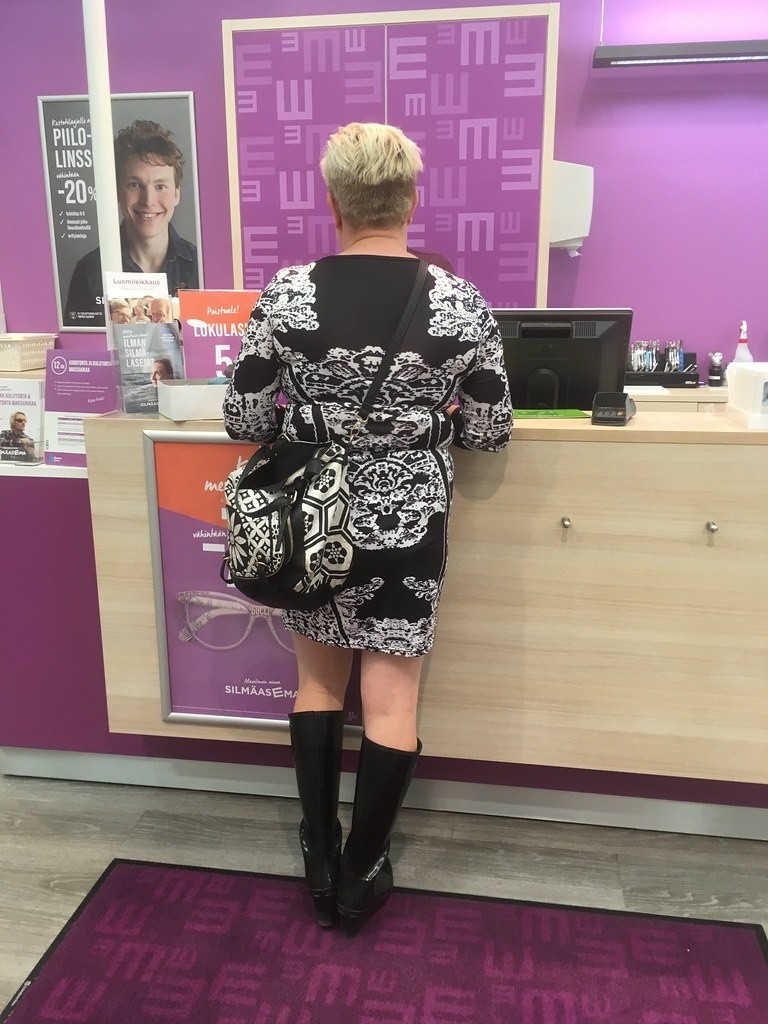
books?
[113,322,185,414]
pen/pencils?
[684,363,698,372]
[664,339,683,372]
[630,340,660,372]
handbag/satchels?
[220,440,354,611]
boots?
[289,710,343,927]
[336,730,422,917]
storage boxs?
[157,377,232,421]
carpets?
[0,857,767,1024]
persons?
[108,295,171,324]
[150,358,174,389]
[222,121,513,939]
[0,412,34,462]
[63,120,199,326]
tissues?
[548,161,594,256]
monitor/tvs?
[490,308,633,411]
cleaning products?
[727,320,754,362]
